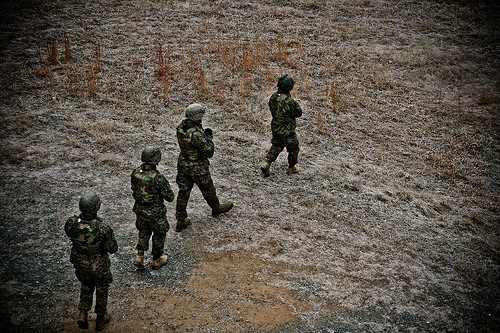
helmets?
[79,193,101,213]
[141,148,161,164]
[185,103,205,121]
[277,74,294,89]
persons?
[64,191,118,331]
[175,104,233,231]
[260,75,303,177]
[130,146,174,269]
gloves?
[204,128,212,136]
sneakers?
[151,255,168,269]
[176,218,191,232]
[95,314,110,328]
[212,202,233,217]
[133,255,144,267]
[77,310,89,330]
[287,167,301,175]
[261,162,271,177]
